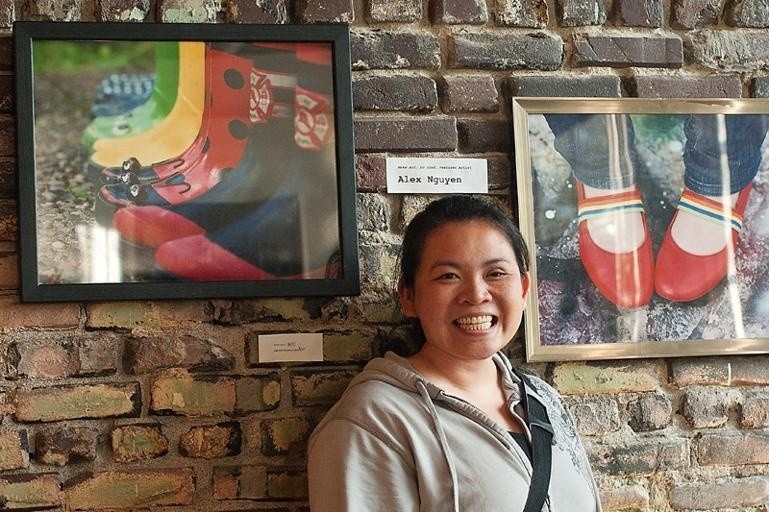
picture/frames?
[506,92,769,367]
[9,19,357,306]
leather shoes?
[655,183,752,303]
[576,180,655,307]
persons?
[306,196,601,512]
[540,114,769,307]
